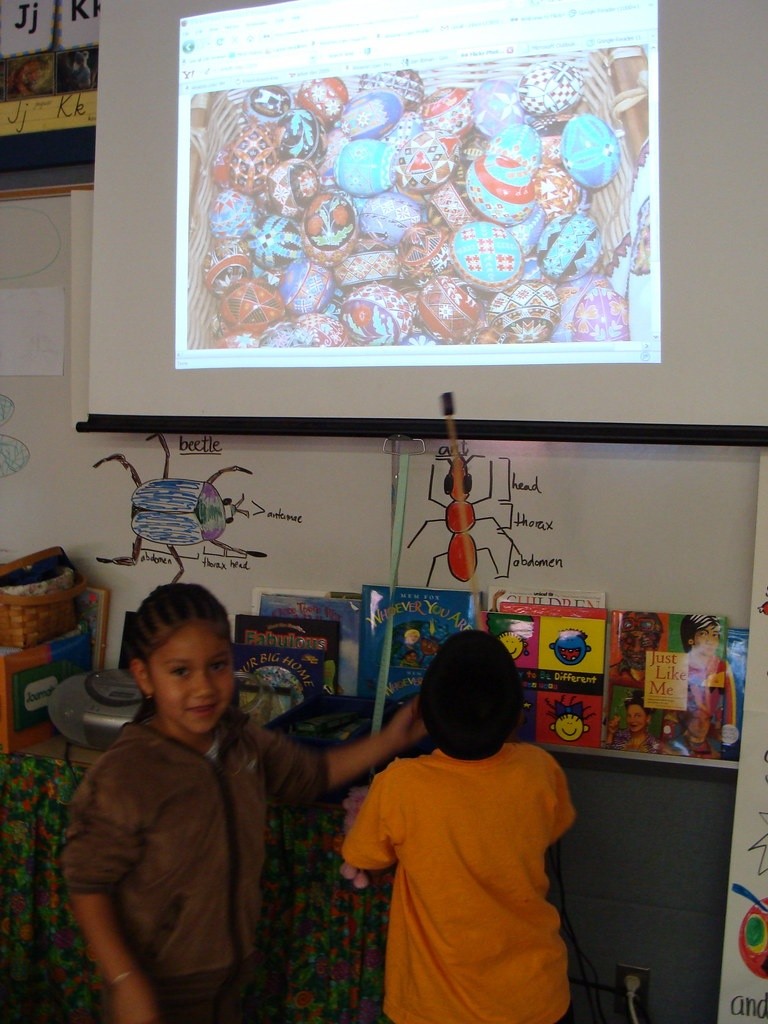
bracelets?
[105,969,137,989]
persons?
[341,629,576,1024]
[63,582,430,1023]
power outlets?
[613,964,651,1019]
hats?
[417,629,524,761]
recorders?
[48,667,143,753]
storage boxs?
[0,631,93,754]
[262,693,395,804]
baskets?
[0,546,90,648]
